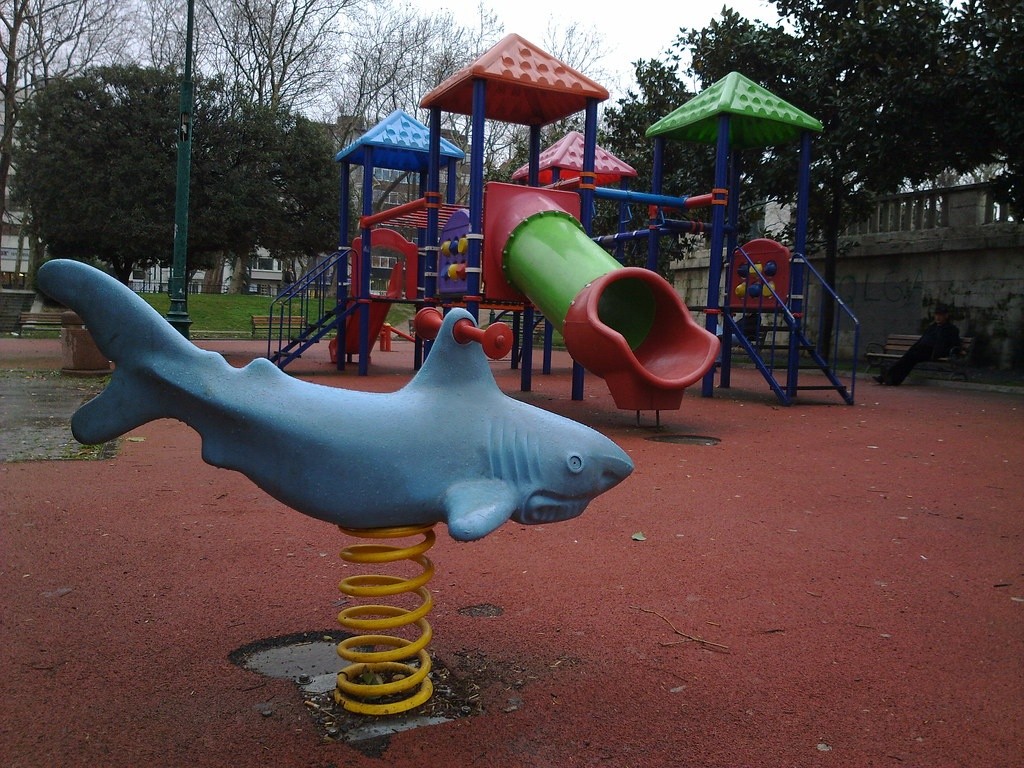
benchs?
[510,320,545,343]
[251,315,307,338]
[864,333,978,382]
[731,329,767,368]
[15,312,63,336]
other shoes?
[718,336,723,342]
[715,362,721,367]
[871,376,899,385]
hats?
[932,305,949,314]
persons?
[871,306,960,387]
[281,267,295,298]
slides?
[326,264,404,363]
[495,193,720,411]
